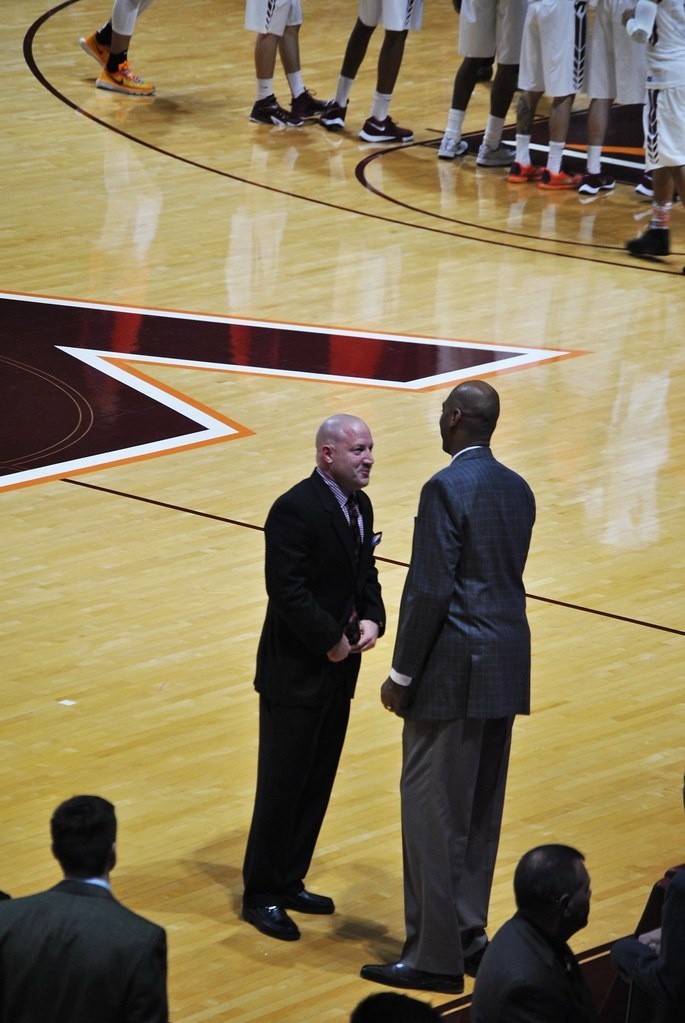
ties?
[347,499,362,541]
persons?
[240,413,389,941]
[244,0,333,127]
[0,795,169,1023]
[319,0,424,143]
[350,992,444,1023]
[469,842,599,1023]
[437,0,685,257]
[610,776,685,1023]
[359,379,537,994]
[80,0,156,97]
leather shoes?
[464,942,490,977]
[360,961,465,994]
[243,903,301,941]
[286,890,335,914]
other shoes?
[627,228,671,257]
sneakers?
[95,60,156,95]
[673,192,681,202]
[319,102,347,129]
[291,87,348,121]
[476,144,517,166]
[540,168,583,190]
[249,94,304,127]
[577,171,616,194]
[79,31,112,67]
[635,174,655,197]
[438,136,468,157]
[508,162,546,183]
[359,116,414,143]
